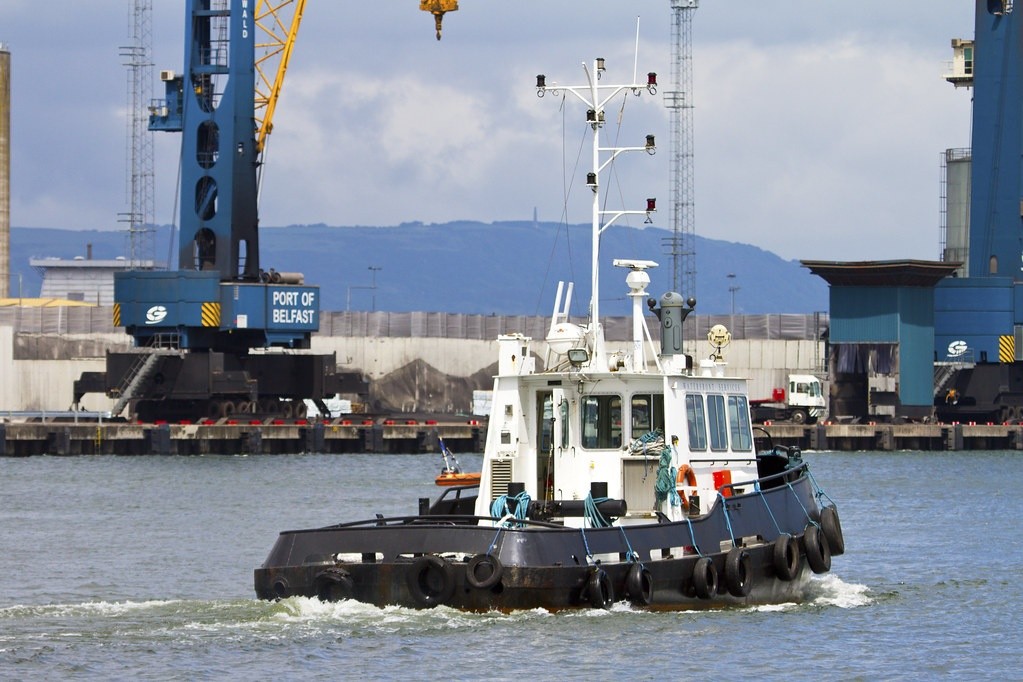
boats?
[254,57,847,613]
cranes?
[255,1,459,153]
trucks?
[749,374,827,424]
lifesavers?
[676,464,697,510]
